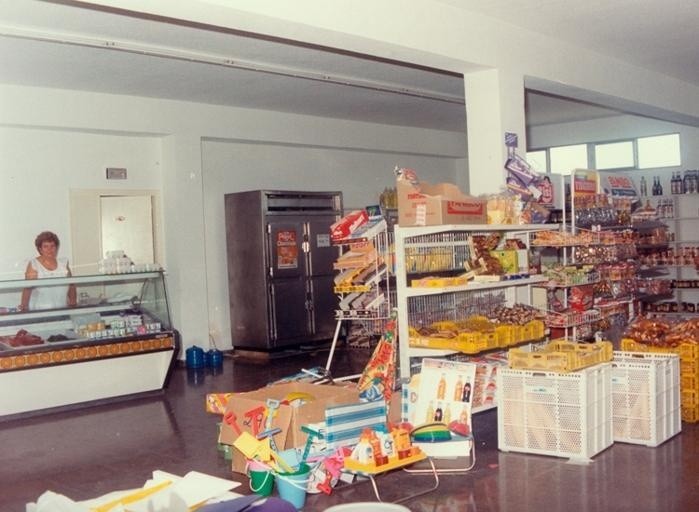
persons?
[18,232,77,315]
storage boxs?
[621,336,698,423]
[610,351,684,447]
[218,380,359,479]
[394,177,489,226]
[498,358,612,458]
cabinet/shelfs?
[0,270,177,420]
[572,224,676,350]
[224,188,350,352]
[334,170,569,419]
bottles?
[672,168,698,198]
[656,177,663,195]
[652,179,660,196]
[640,176,647,195]
[654,199,674,222]
